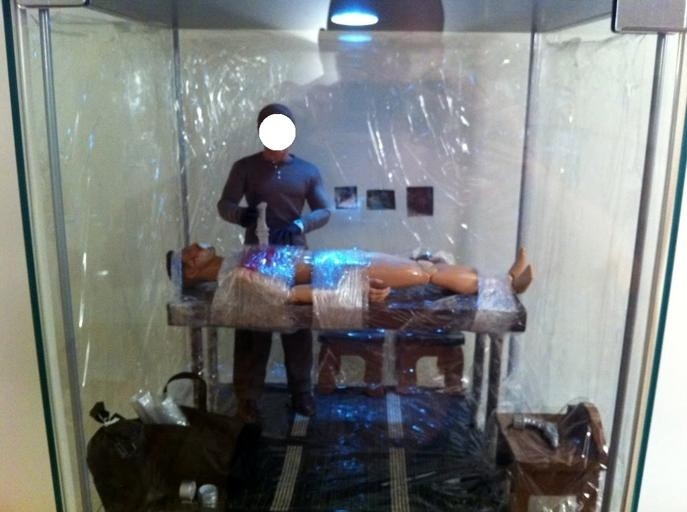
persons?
[255,1,490,130]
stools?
[315,328,470,399]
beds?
[168,284,528,438]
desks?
[494,399,609,512]
